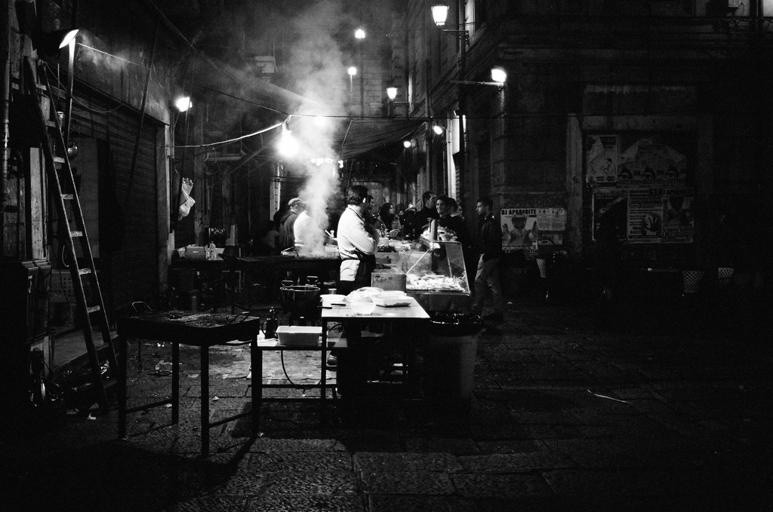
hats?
[288,198,303,205]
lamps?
[56,26,80,49]
[430,0,470,46]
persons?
[616,169,633,184]
[584,133,608,182]
[643,214,660,235]
[502,214,537,246]
[269,185,512,326]
[653,127,738,331]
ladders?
[25,57,118,414]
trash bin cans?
[423,308,497,402]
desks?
[320,292,432,438]
[255,325,348,432]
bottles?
[209,241,216,259]
[261,307,279,338]
